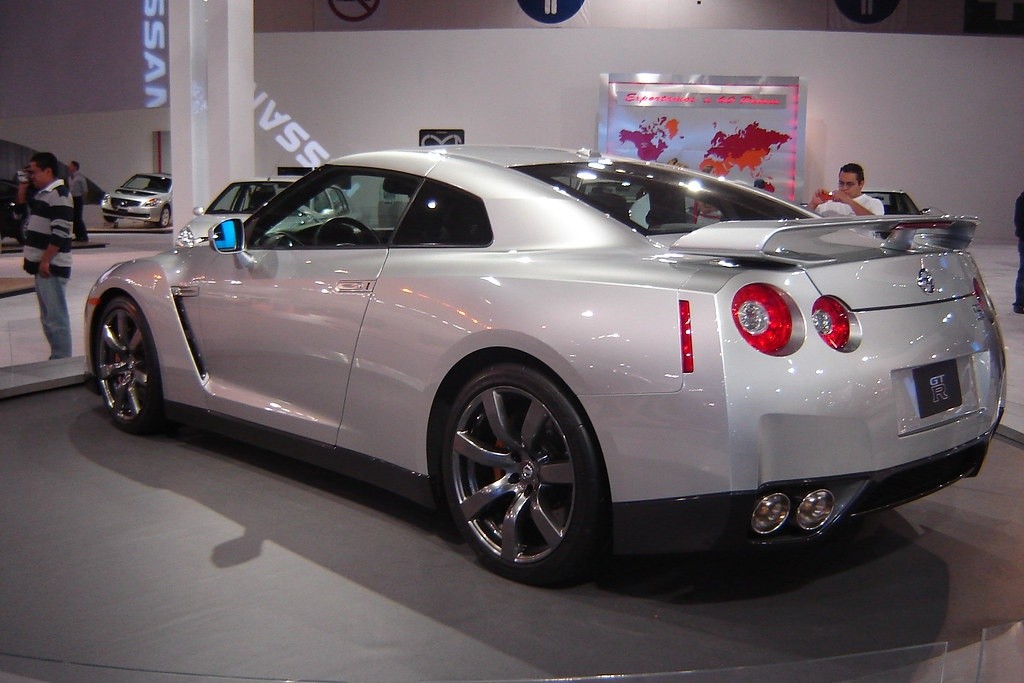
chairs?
[589,191,627,214]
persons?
[16,166,31,221]
[67,161,89,241]
[22,152,73,359]
[1013,190,1024,313]
[807,163,884,236]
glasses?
[838,182,858,187]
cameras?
[16,170,32,183]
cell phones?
[820,192,833,200]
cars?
[99,173,173,229]
[802,190,931,218]
[175,174,363,248]
[0,178,30,244]
[79,144,1009,583]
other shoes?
[1013,305,1024,314]
